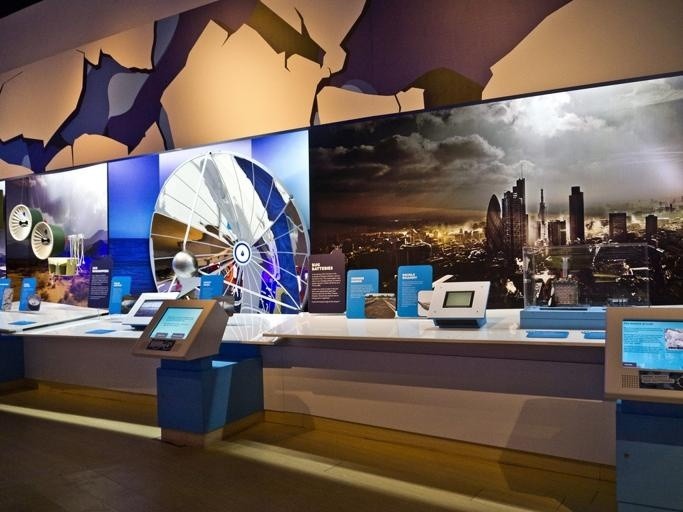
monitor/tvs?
[130,299,229,360]
[122,292,180,331]
[603,307,683,404]
